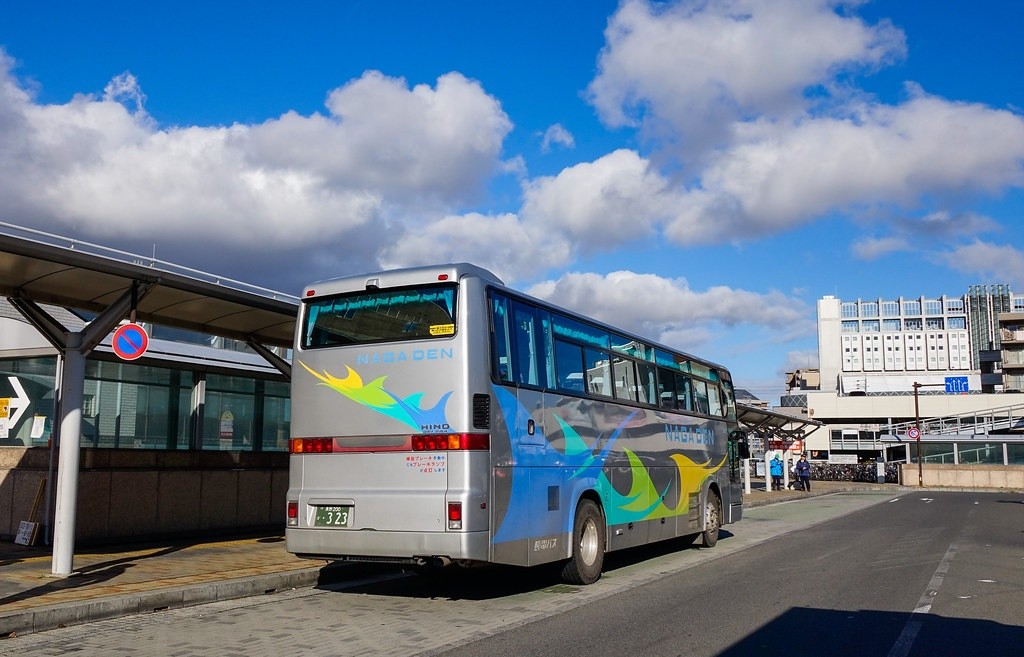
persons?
[770,454,782,491]
[796,453,810,492]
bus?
[282,262,748,586]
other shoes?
[773,484,811,492]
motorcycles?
[782,461,898,484]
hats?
[774,454,806,460]
